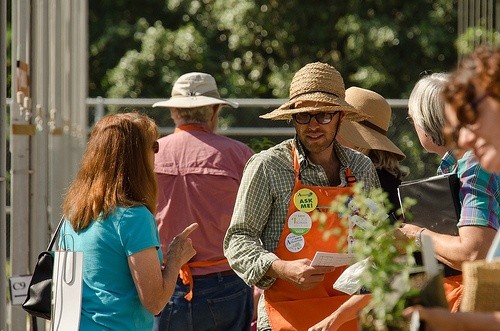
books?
[397,174,462,237]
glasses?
[448,93,490,133]
[150,141,159,153]
[406,116,414,125]
[291,111,338,125]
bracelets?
[415,229,422,248]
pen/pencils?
[391,212,405,228]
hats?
[337,86,406,159]
[259,62,367,121]
[152,72,239,108]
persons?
[335,86,406,227]
[59,112,199,331]
[151,72,256,331]
[307,46,500,331]
[222,62,381,331]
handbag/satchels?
[21,214,65,320]
